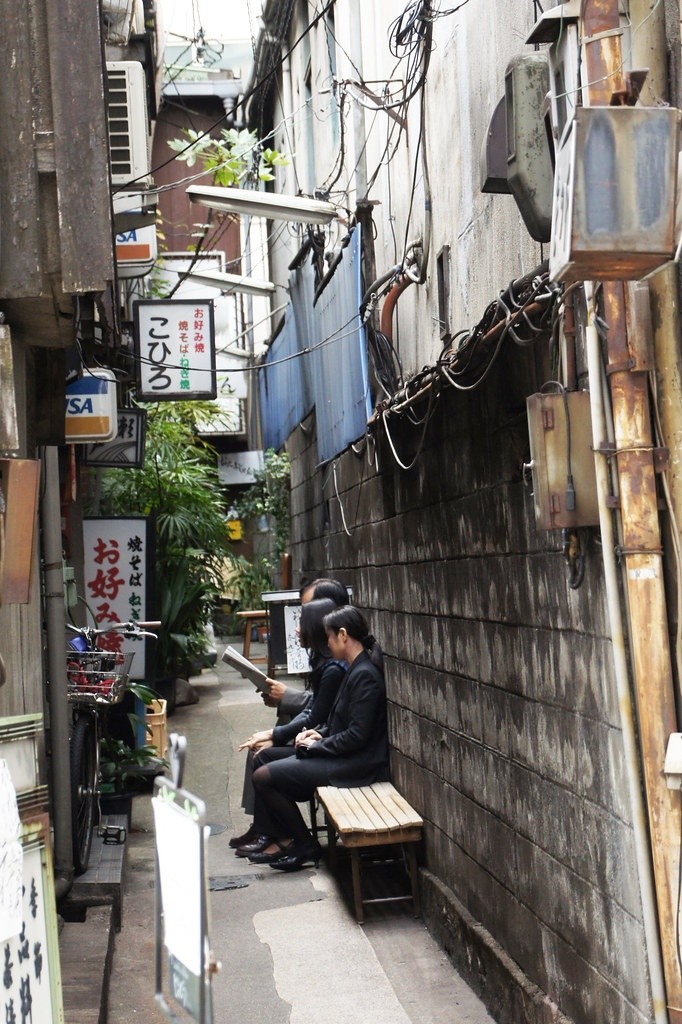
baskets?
[144,697,168,762]
[43,645,134,706]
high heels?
[249,837,297,861]
[269,840,323,872]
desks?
[236,609,269,677]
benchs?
[310,781,423,925]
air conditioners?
[106,59,151,189]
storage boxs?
[144,698,167,758]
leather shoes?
[235,834,268,857]
[229,825,263,847]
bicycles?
[62,618,163,874]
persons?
[240,604,387,876]
[234,598,351,862]
[229,577,350,858]
